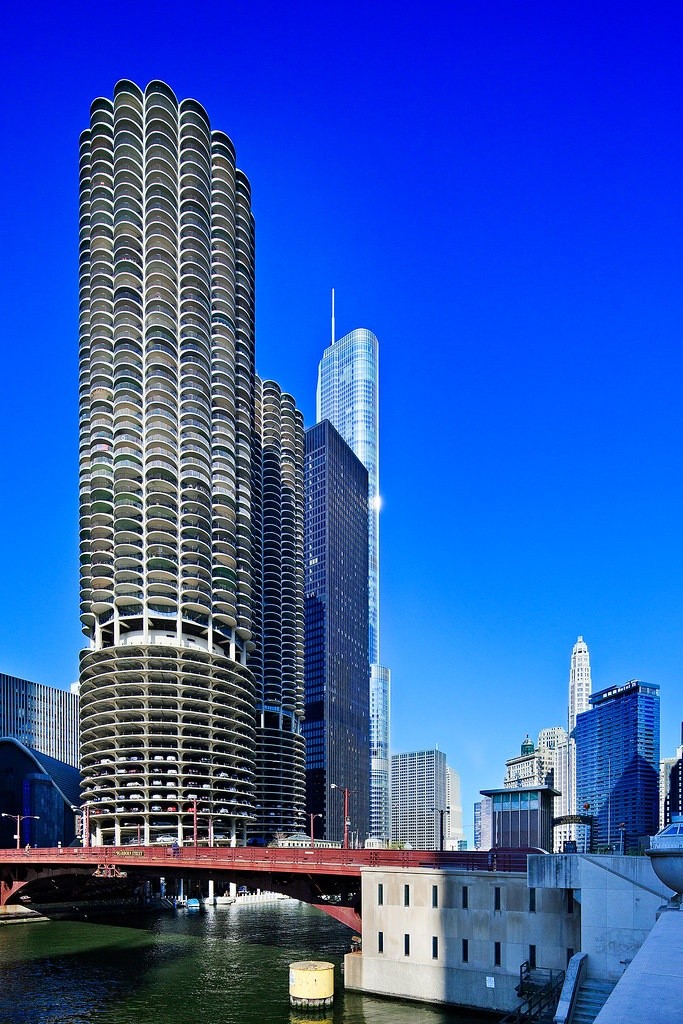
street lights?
[1,812,41,849]
[297,812,323,848]
[329,782,361,850]
[430,806,450,852]
[197,816,222,846]
[70,804,90,848]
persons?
[25,843,31,857]
[172,839,179,858]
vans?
[488,846,551,872]
